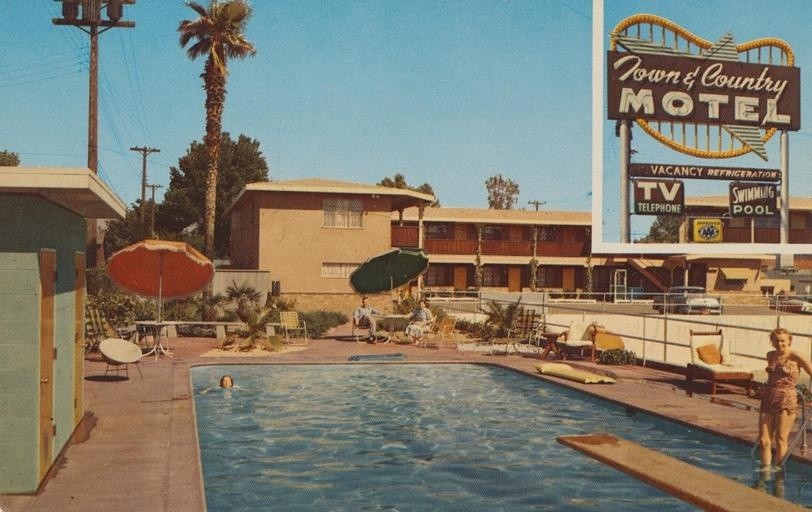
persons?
[199,374,245,396]
[734,466,787,503]
[406,301,436,345]
[751,328,812,471]
[354,295,382,344]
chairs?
[538,321,573,360]
[352,310,377,343]
[687,330,754,403]
[491,309,544,360]
[565,321,596,361]
[99,338,144,382]
[279,311,307,346]
[412,313,457,351]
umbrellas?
[102,236,218,324]
[347,247,432,316]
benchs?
[263,323,280,336]
[163,321,245,339]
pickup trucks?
[653,284,722,316]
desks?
[135,321,185,363]
[377,315,410,344]
